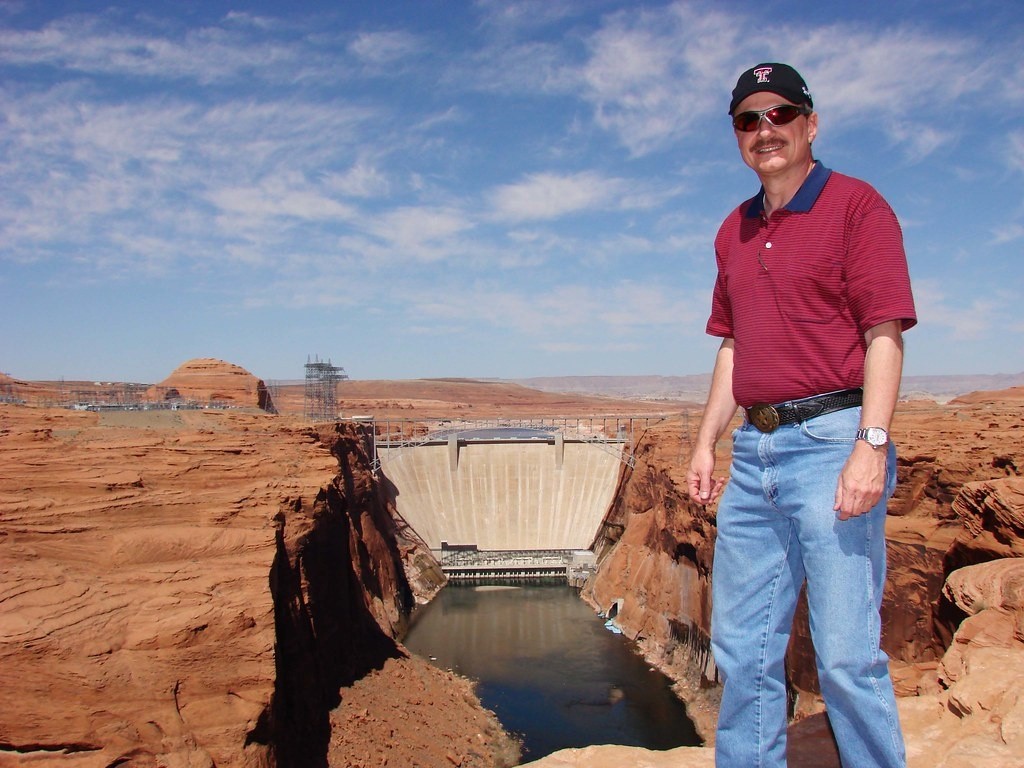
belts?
[742,387,863,433]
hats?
[728,63,813,115]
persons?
[686,62,917,768]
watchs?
[855,427,890,450]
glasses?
[732,102,811,132]
